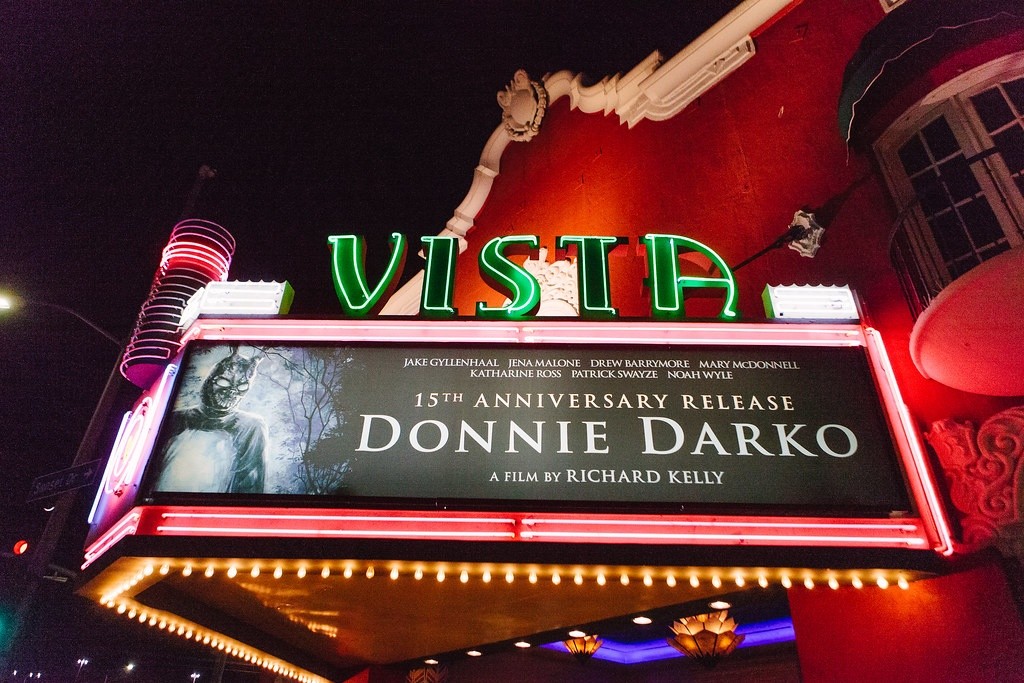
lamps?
[563,635,604,664]
[667,610,746,671]
